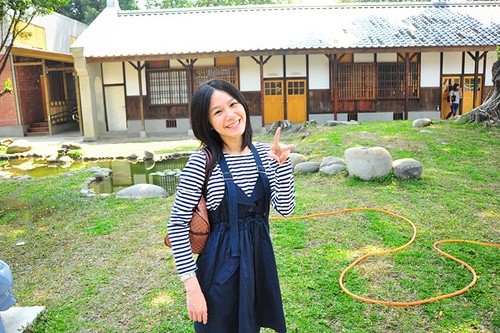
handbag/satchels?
[447,90,455,102]
[164,148,210,254]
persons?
[166,76,296,333]
[446,83,462,119]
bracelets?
[181,271,196,282]
